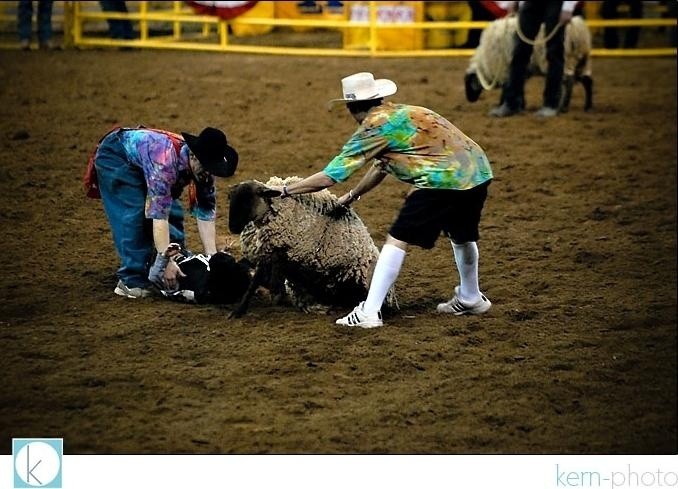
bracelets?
[349,189,362,202]
[282,186,288,195]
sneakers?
[488,102,513,116]
[437,284,492,315]
[335,301,384,329]
[114,278,151,299]
[533,106,556,117]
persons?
[266,71,495,329]
[599,0,641,47]
[489,1,580,117]
[17,0,53,49]
[147,242,254,305]
[100,0,135,51]
[83,124,239,301]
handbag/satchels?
[82,127,119,198]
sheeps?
[223,173,404,321]
[461,12,595,113]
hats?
[206,253,250,304]
[327,72,397,112]
[181,127,238,177]
